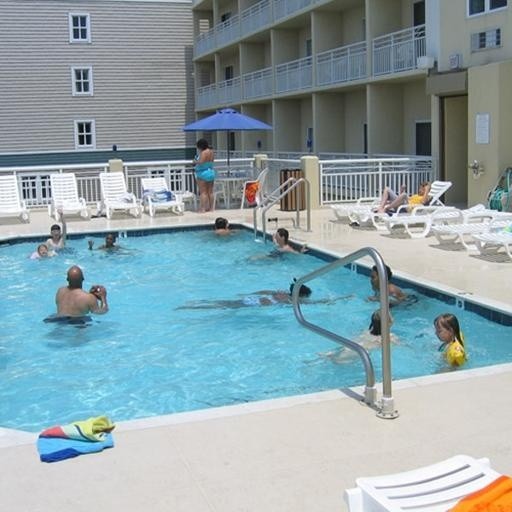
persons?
[173,276,356,309]
[193,138,216,214]
[87,232,134,258]
[246,228,310,264]
[214,216,232,236]
[370,182,432,214]
[37,243,57,259]
[433,313,468,372]
[313,308,402,364]
[363,263,424,315]
[44,207,67,251]
[42,265,109,346]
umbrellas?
[181,108,275,210]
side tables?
[174,190,197,211]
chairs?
[0,171,180,224]
[330,180,512,263]
[212,165,269,209]
